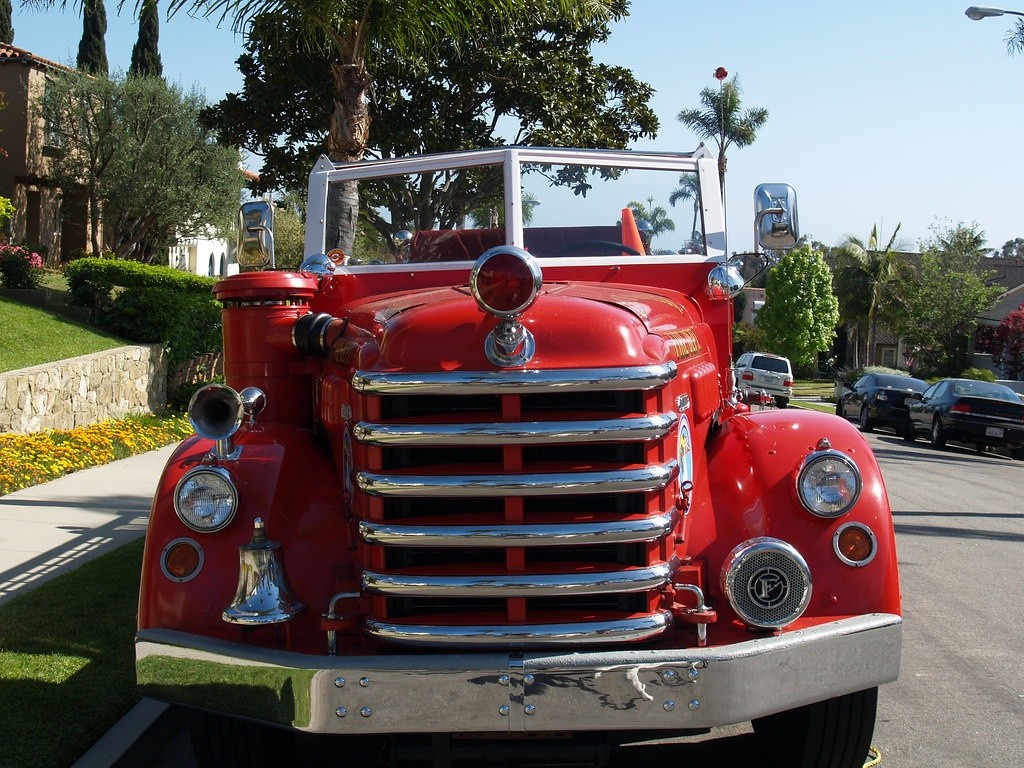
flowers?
[0,241,48,290]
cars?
[904,378,1024,458]
[133,144,901,768]
[835,373,930,442]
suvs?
[733,351,793,408]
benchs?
[410,226,623,264]
